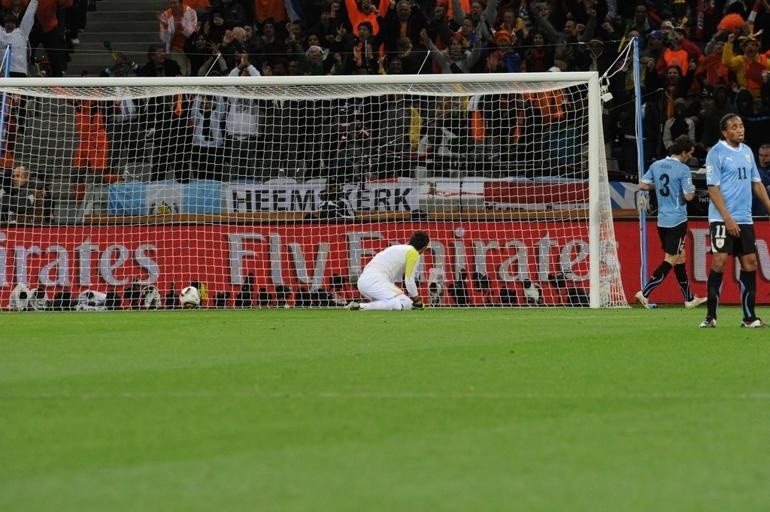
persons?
[347,231,429,310]
[0,1,770,215]
[634,133,707,309]
[694,112,770,327]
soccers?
[180,285,201,306]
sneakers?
[740,318,766,328]
[700,318,716,327]
[634,291,653,309]
[684,295,708,309]
